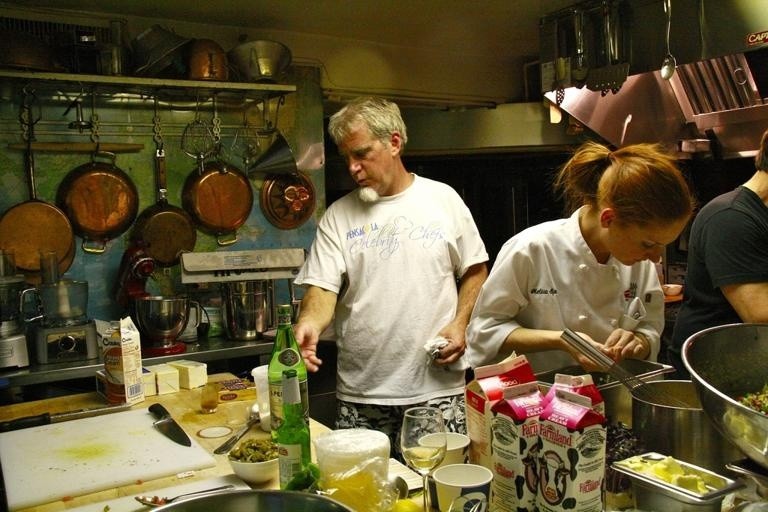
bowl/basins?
[681,321,767,469]
[130,23,198,80]
[229,39,292,81]
[227,451,277,485]
[663,283,682,296]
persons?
[288,93,490,480]
[664,130,766,381]
[462,143,694,369]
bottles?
[268,303,311,442]
[276,369,312,492]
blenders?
[111,246,189,357]
[17,280,100,365]
[0,250,29,370]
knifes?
[148,403,191,448]
[213,419,261,454]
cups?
[421,431,470,506]
[318,429,388,511]
[432,461,494,512]
[251,362,273,431]
[202,384,220,411]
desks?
[1,372,440,512]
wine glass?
[400,407,446,511]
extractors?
[666,52,757,116]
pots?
[0,141,78,283]
[630,378,751,484]
[181,149,254,247]
[57,150,139,253]
[134,149,196,267]
[145,488,356,510]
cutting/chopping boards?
[1,408,218,511]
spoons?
[139,483,234,507]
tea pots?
[179,301,200,343]
